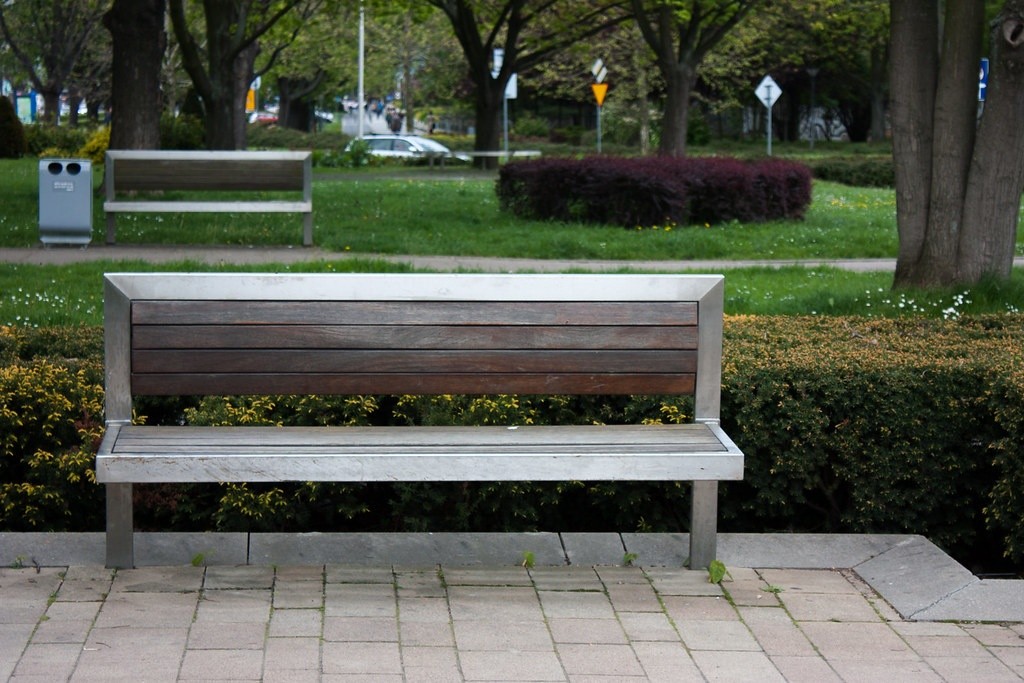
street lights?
[805,59,822,149]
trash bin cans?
[36,157,95,250]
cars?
[343,132,472,167]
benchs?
[103,150,314,247]
[414,150,542,170]
[94,272,749,567]
